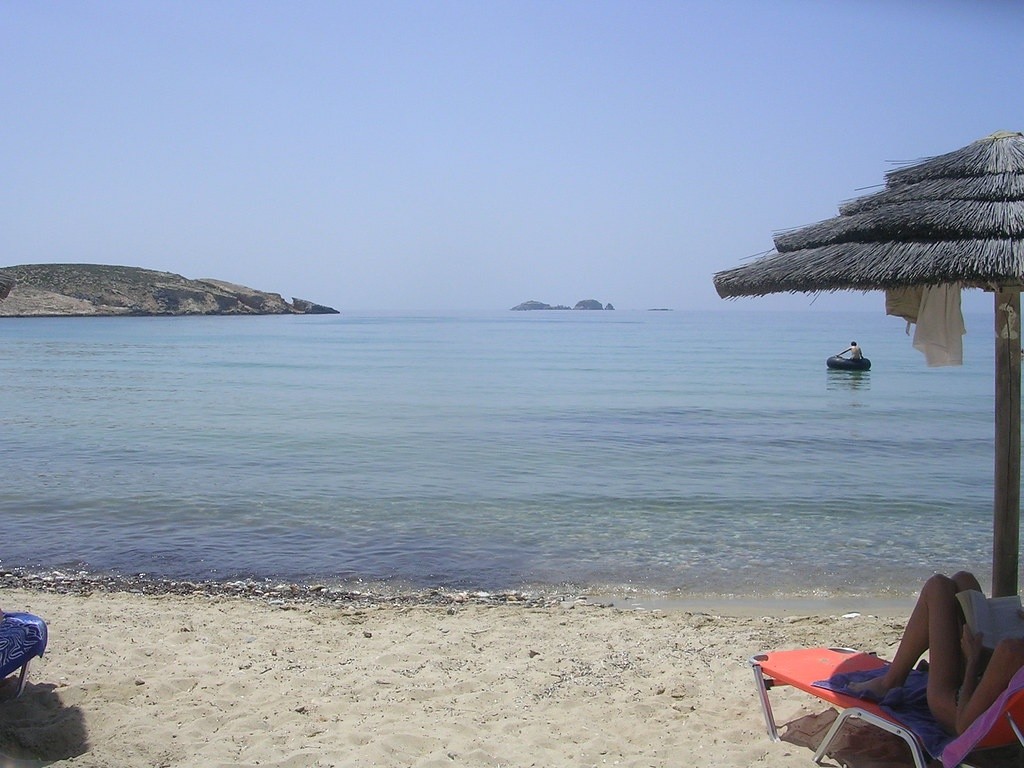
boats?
[826,355,872,372]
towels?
[942,665,1024,768]
[886,286,923,336]
[912,283,967,368]
[811,663,956,761]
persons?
[839,342,863,360]
[849,572,1024,735]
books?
[955,589,1024,648]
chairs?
[0,610,48,705]
[750,647,1024,768]
